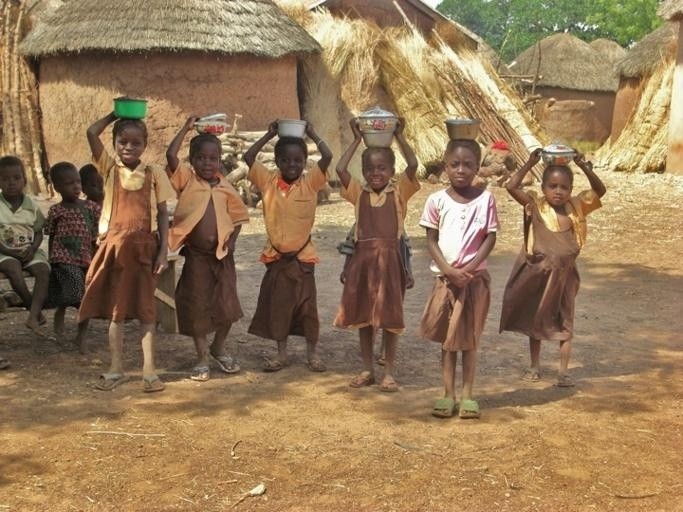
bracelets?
[315,140,322,147]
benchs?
[0,252,186,342]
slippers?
[556,374,575,388]
[259,355,289,371]
[305,359,326,372]
[348,369,374,388]
[189,366,210,381]
[458,399,480,419]
[207,350,240,373]
[430,398,456,418]
[142,376,164,392]
[520,367,543,382]
[94,372,129,391]
[379,378,397,392]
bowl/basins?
[275,119,306,139]
[443,119,481,140]
[193,120,227,135]
[539,145,576,166]
[113,98,148,120]
[357,106,397,148]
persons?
[418,138,502,418]
[75,94,170,392]
[242,118,333,371]
[44,161,98,356]
[78,163,104,219]
[0,155,51,338]
[497,146,606,390]
[337,215,414,366]
[335,116,418,392]
[164,114,250,382]
